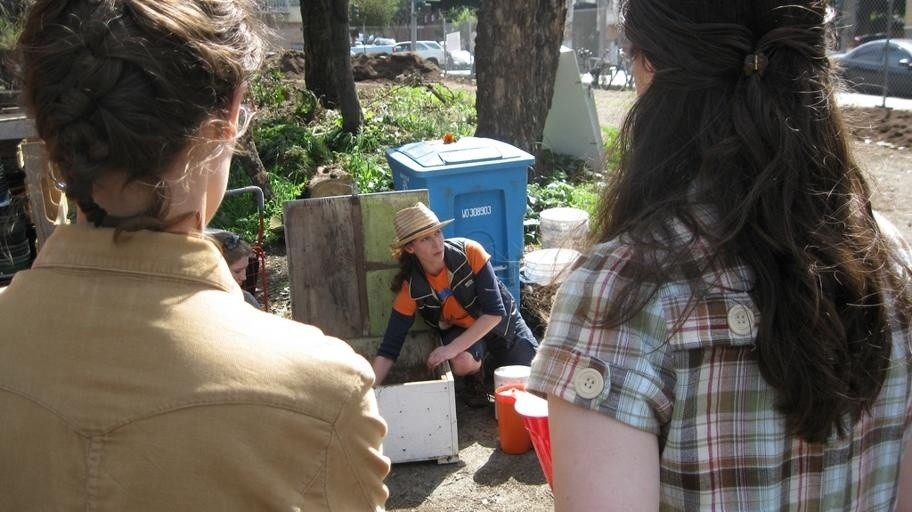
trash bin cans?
[386,136,535,313]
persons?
[205,229,251,290]
[371,201,540,408]
[523,0,912,512]
[0,0,391,512]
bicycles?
[584,48,635,89]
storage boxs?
[282,330,459,464]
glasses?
[222,233,240,253]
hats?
[389,202,456,249]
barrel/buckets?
[492,363,533,423]
[485,384,534,458]
[513,392,554,499]
[538,206,590,256]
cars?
[832,36,911,93]
[350,38,475,70]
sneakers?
[463,365,489,406]
